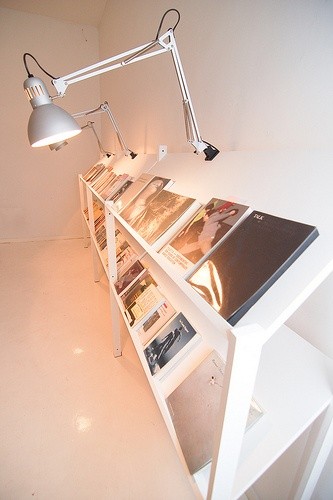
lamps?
[23,8,220,162]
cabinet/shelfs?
[78,152,333,500]
